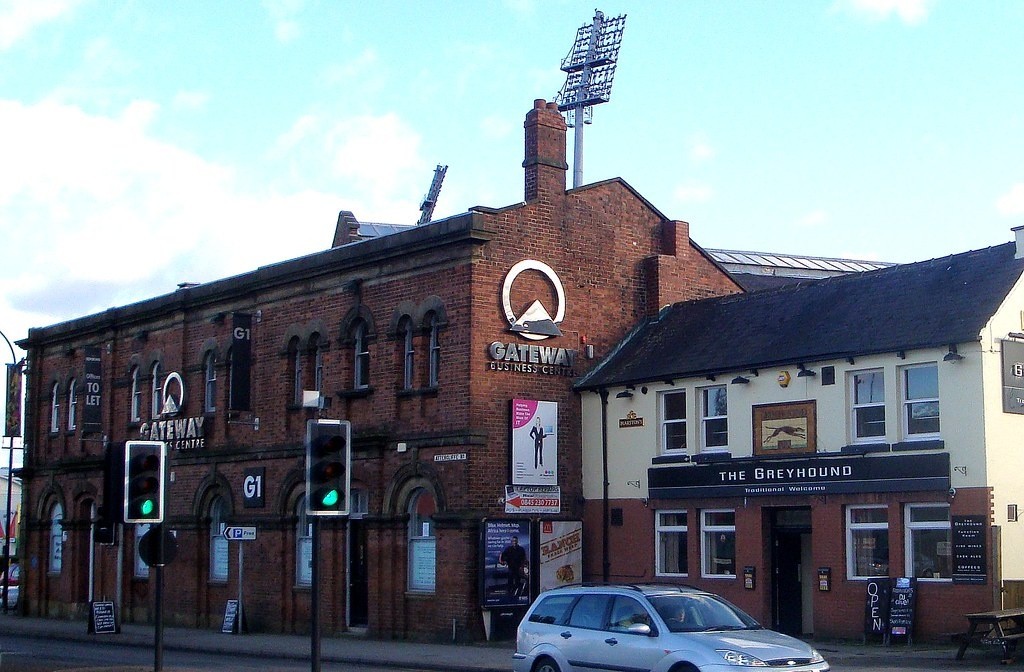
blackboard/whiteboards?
[951,515,986,575]
[93,602,116,633]
[885,577,916,635]
[864,578,890,633]
[221,599,239,634]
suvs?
[513,580,831,672]
[0,563,19,608]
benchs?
[951,630,988,644]
[999,633,1024,663]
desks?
[955,609,1024,664]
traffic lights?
[123,441,166,525]
[305,417,352,518]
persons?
[530,417,547,469]
[499,535,526,594]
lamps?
[845,356,854,365]
[797,362,816,377]
[616,383,648,398]
[943,343,963,365]
[731,368,758,384]
[897,351,905,360]
[665,379,675,386]
[706,373,716,381]
